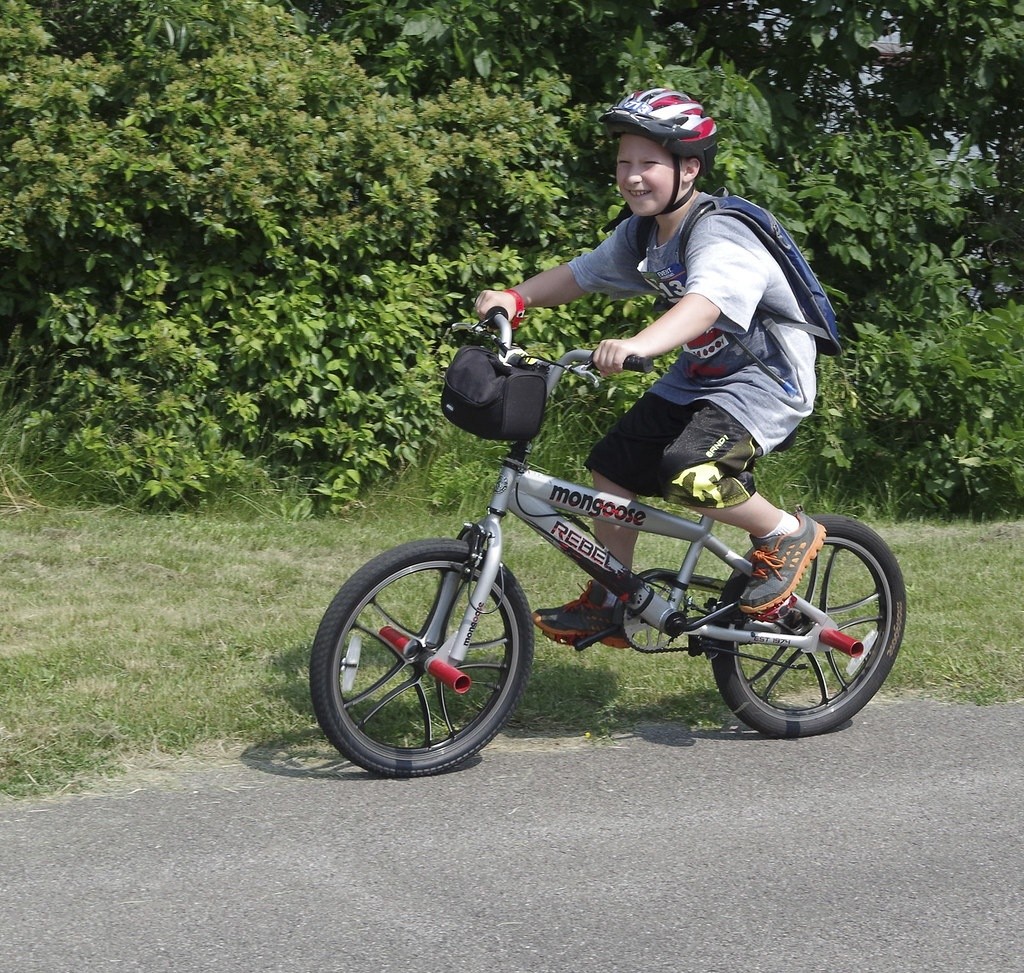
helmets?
[600,85,716,175]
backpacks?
[635,188,843,355]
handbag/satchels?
[440,348,546,442]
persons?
[487,82,837,654]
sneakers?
[739,505,828,612]
[534,580,633,649]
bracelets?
[502,285,527,331]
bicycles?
[309,306,909,778]
